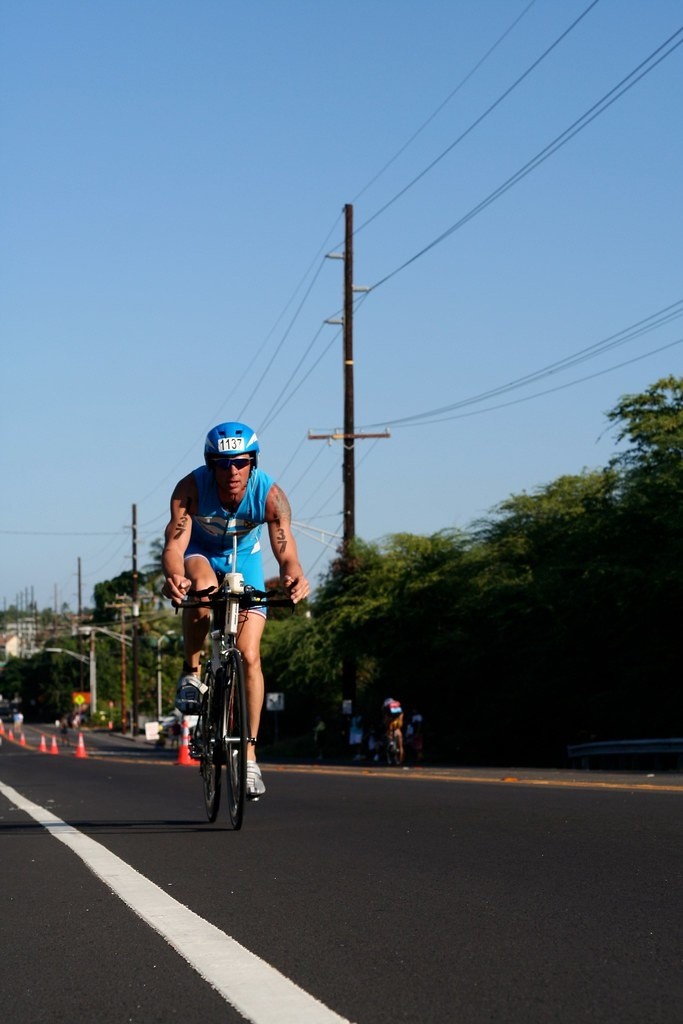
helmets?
[204,422,260,471]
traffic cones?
[19,731,25,746]
[74,732,90,758]
[48,734,59,755]
[0,717,5,735]
[174,719,201,766]
[8,727,14,741]
[39,733,49,753]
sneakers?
[176,675,208,711]
[246,761,266,796]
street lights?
[45,646,97,721]
[157,629,175,722]
[78,625,139,737]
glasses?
[209,458,254,470]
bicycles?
[171,582,304,829]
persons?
[13,709,23,734]
[162,422,310,798]
[59,703,87,747]
[348,698,421,763]
[156,720,181,748]
[314,716,326,760]
